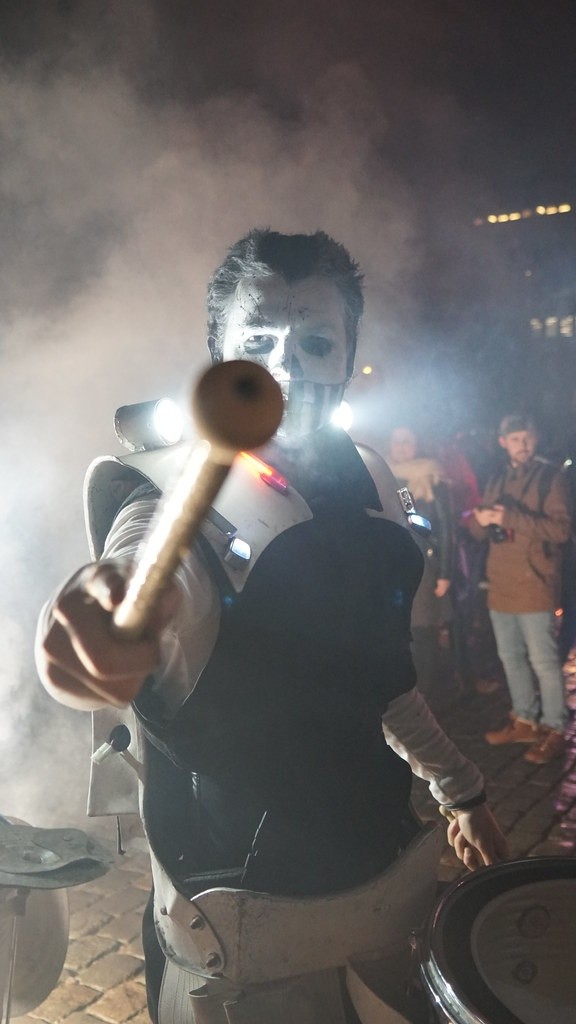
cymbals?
[1,815,116,889]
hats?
[501,414,537,435]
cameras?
[477,504,511,541]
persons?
[463,411,574,764]
[365,421,575,717]
[36,228,511,1024]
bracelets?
[444,789,487,811]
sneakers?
[525,724,565,764]
[486,713,539,744]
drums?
[406,853,576,1024]
[0,815,70,1019]
